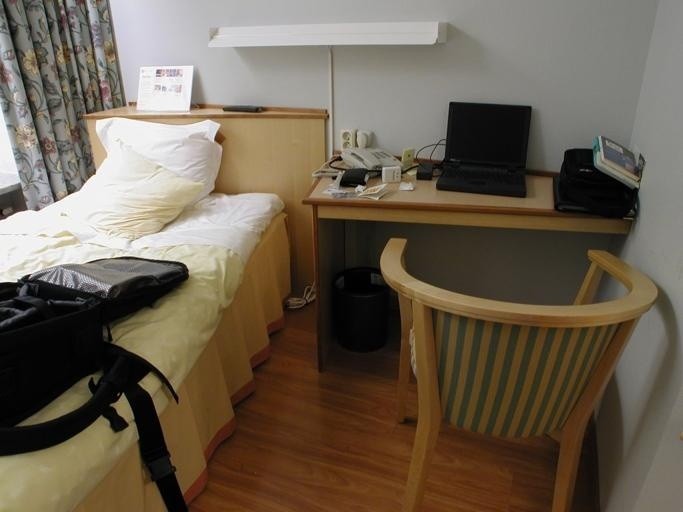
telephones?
[341,147,402,177]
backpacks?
[0,255,192,512]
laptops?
[435,101,531,198]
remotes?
[222,106,260,113]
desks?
[302,156,633,373]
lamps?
[208,21,447,47]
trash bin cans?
[333,266,394,352]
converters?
[416,162,434,181]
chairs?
[376,236,659,512]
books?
[592,133,642,190]
[359,182,392,201]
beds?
[0,95,331,510]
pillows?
[73,118,225,244]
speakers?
[340,128,358,152]
